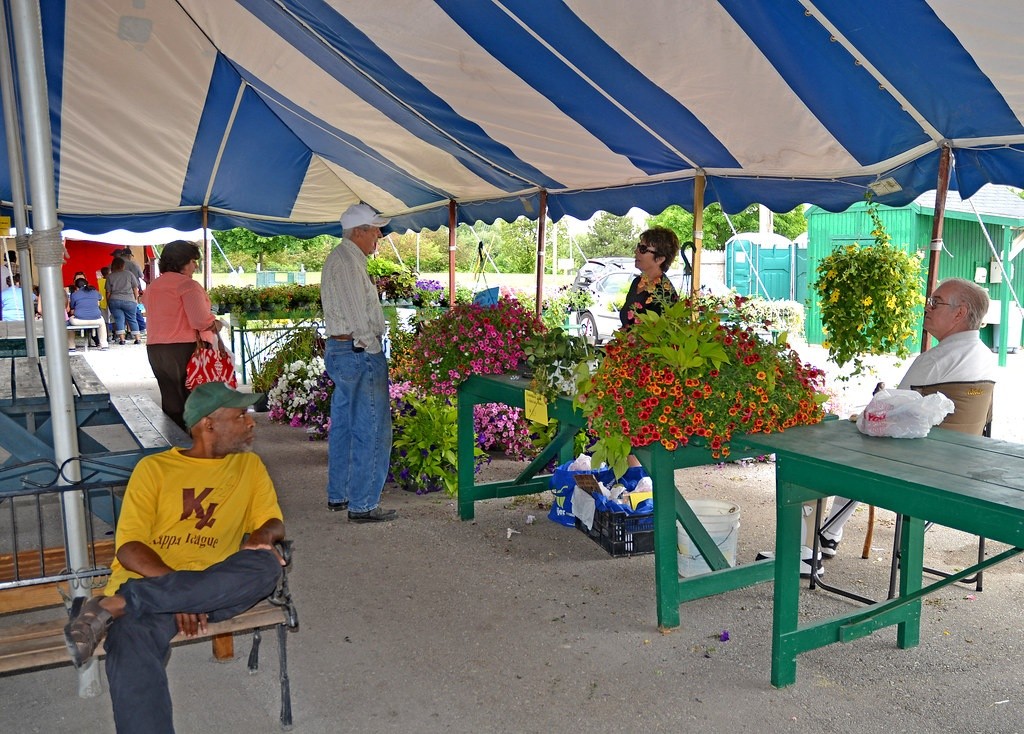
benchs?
[107,393,193,454]
[0,457,299,731]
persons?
[143,240,223,430]
[620,227,681,466]
[322,205,398,522]
[756,277,997,579]
[67,271,109,351]
[1,250,38,321]
[96,248,146,344]
[63,382,285,734]
[38,291,70,326]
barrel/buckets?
[675,499,741,577]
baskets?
[572,498,655,557]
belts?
[330,334,353,341]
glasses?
[926,296,954,309]
[634,242,658,256]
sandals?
[66,593,118,669]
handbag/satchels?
[854,386,958,438]
[187,326,244,390]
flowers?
[206,253,598,499]
[803,183,935,389]
[575,260,830,461]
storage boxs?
[573,482,656,558]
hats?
[184,380,265,427]
[110,248,125,256]
[339,202,392,229]
[121,248,135,258]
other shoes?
[100,347,107,351]
[817,534,840,559]
[755,544,827,581]
[327,501,350,512]
[134,340,144,344]
[68,349,76,352]
[118,340,126,345]
[346,508,399,524]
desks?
[66,325,99,352]
[0,319,46,355]
[733,416,1024,690]
[451,371,841,628]
[0,353,123,528]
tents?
[0,227,244,322]
[0,0,1024,693]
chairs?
[809,380,998,607]
